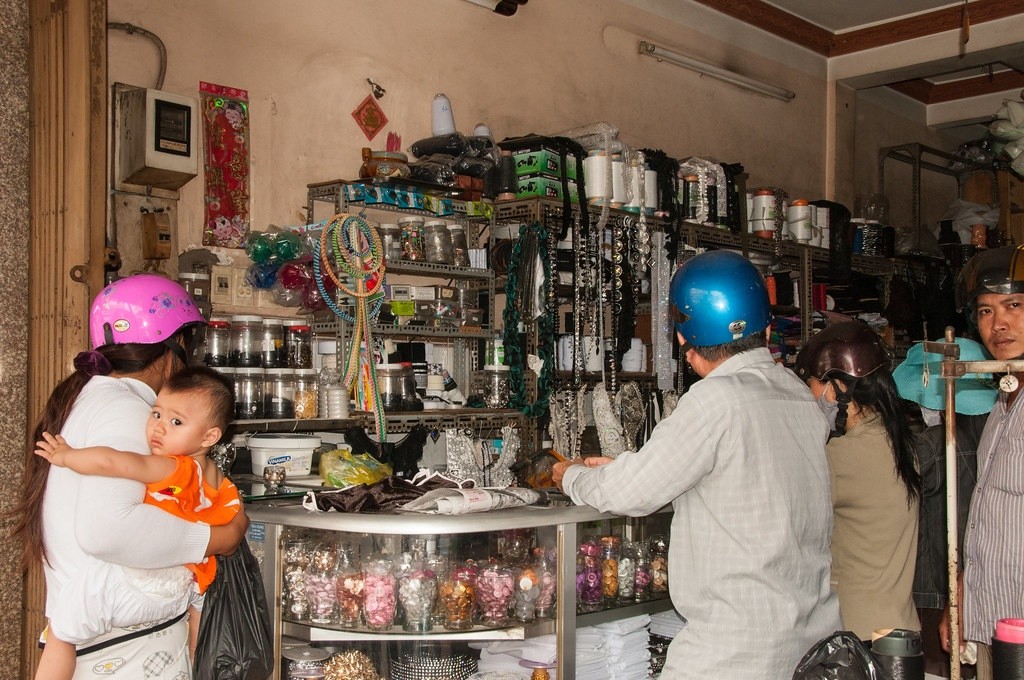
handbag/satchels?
[192,534,274,680]
[791,631,877,680]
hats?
[892,337,1001,415]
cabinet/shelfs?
[205,142,999,482]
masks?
[817,378,839,431]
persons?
[803,318,922,653]
[6,273,253,680]
[550,249,838,680]
[938,245,1024,679]
[35,365,241,679]
[893,336,1003,679]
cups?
[282,534,670,633]
[208,442,236,480]
[263,466,286,490]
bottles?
[424,220,451,264]
[399,216,426,262]
[373,362,417,412]
[206,316,349,419]
[447,224,471,267]
[177,272,211,362]
[483,365,510,409]
[368,221,400,260]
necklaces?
[497,209,651,418]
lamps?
[638,42,797,104]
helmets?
[796,320,890,380]
[670,251,773,346]
[89,275,215,352]
[954,248,1024,314]
